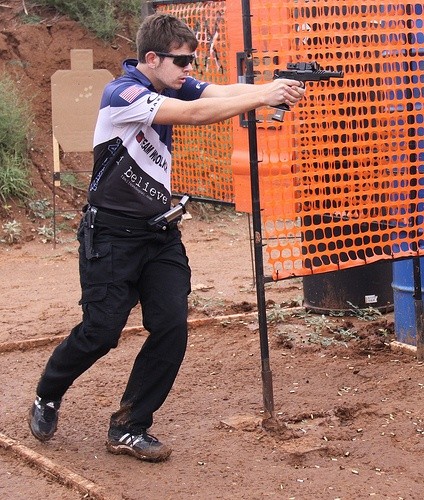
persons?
[29,14,305,460]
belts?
[93,209,151,231]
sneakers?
[30,394,62,442]
[106,425,173,461]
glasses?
[155,50,196,68]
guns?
[269,61,344,111]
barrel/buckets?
[377,1,423,355]
[294,1,394,314]
